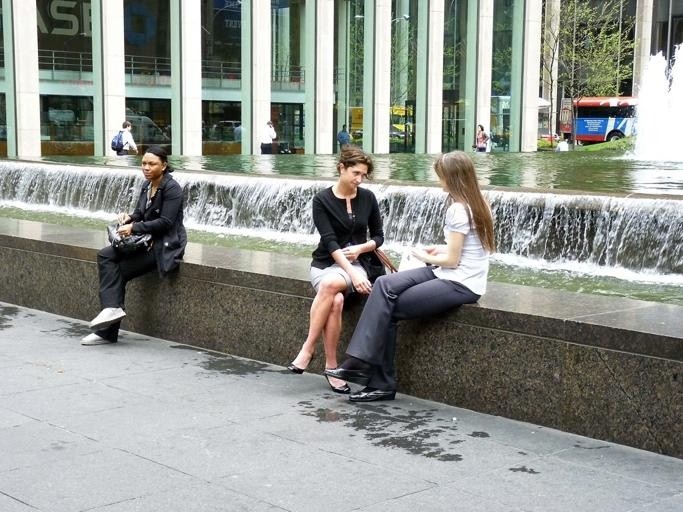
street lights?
[107,219,155,255]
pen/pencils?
[213,120,241,141]
[125,113,170,143]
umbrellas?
[324,368,370,386]
[349,389,396,402]
[324,371,351,394]
[287,355,314,374]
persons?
[555,137,569,151]
[323,149,496,403]
[258,120,277,154]
[116,121,140,156]
[286,142,387,394]
[475,124,490,152]
[80,146,188,346]
[337,124,350,149]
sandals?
[572,94,638,143]
[348,106,412,137]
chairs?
[90,306,127,330]
[82,333,109,345]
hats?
[350,122,409,140]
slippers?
[112,130,128,152]
